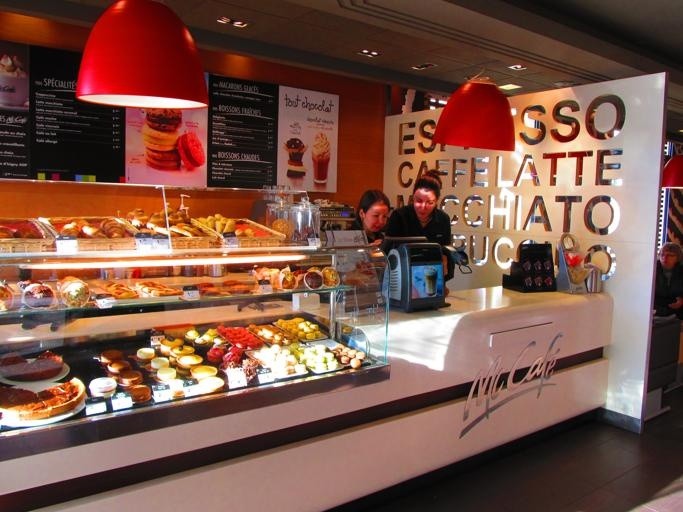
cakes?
[249,316,321,347]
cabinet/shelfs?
[0,246,391,512]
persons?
[653,242,683,319]
[383,170,451,257]
[348,190,390,245]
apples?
[289,342,366,369]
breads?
[60,218,125,239]
[198,214,255,237]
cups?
[261,183,309,204]
[310,130,329,186]
[265,203,321,245]
[422,267,439,297]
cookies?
[142,109,206,171]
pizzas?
[0,377,86,421]
[0,351,64,382]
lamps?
[659,142,683,188]
[432,64,516,151]
[75,0,209,110]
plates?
[218,320,371,383]
